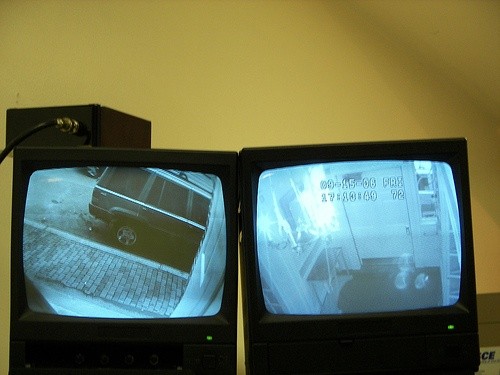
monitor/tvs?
[237,138,484,374]
[12,149,238,374]
